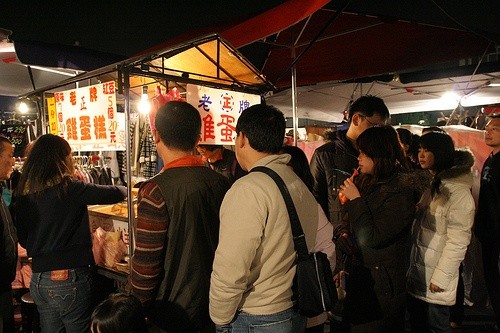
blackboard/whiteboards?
[0,125,27,157]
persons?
[396,128,446,194]
[90,292,144,333]
[11,134,128,333]
[195,144,248,187]
[129,100,231,333]
[310,95,389,246]
[337,125,417,333]
[0,137,18,333]
[405,133,475,333]
[209,104,337,333]
[279,146,341,333]
[474,114,500,333]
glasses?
[360,114,380,128]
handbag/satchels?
[293,251,339,318]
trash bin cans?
[20,291,42,333]
